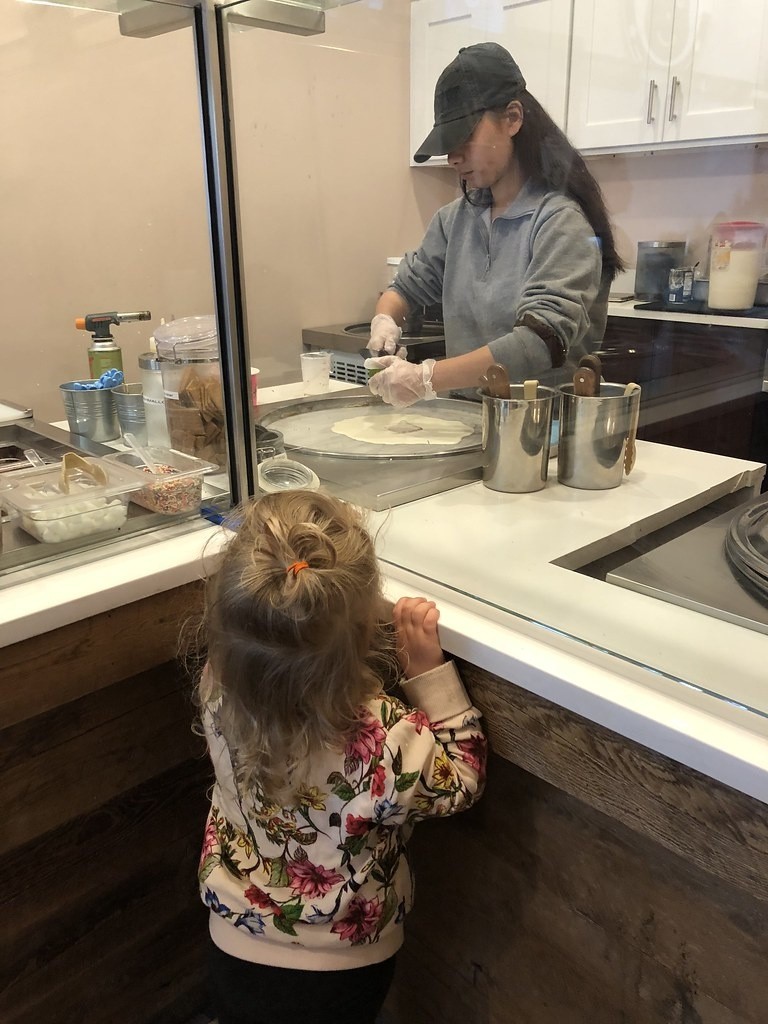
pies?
[332,413,475,445]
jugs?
[709,221,767,309]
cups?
[668,268,694,304]
[248,366,259,409]
[635,241,685,300]
[300,352,331,394]
[140,353,170,448]
[555,379,642,490]
[478,384,554,491]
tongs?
[621,383,640,476]
[60,453,108,492]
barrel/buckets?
[113,383,150,448]
[59,379,122,443]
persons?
[178,489,487,1024]
[364,42,626,407]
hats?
[413,42,526,163]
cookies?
[165,369,228,475]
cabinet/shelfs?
[566,1,768,158]
[600,316,768,496]
[408,0,572,169]
